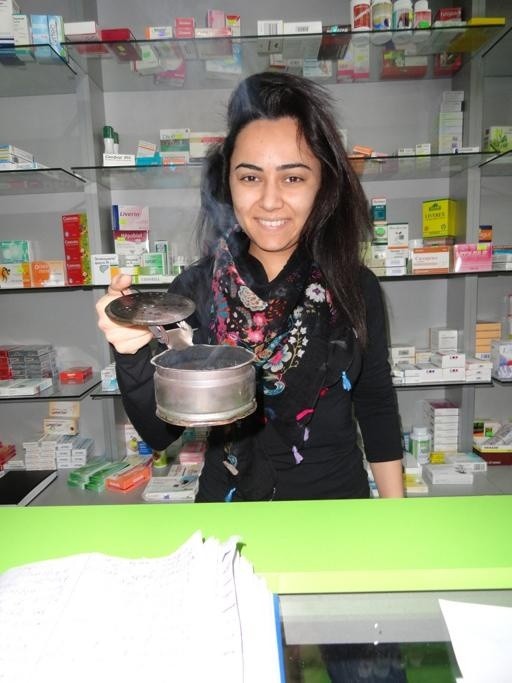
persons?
[97,72,403,503]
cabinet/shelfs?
[2,15,512,501]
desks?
[3,494,509,682]
